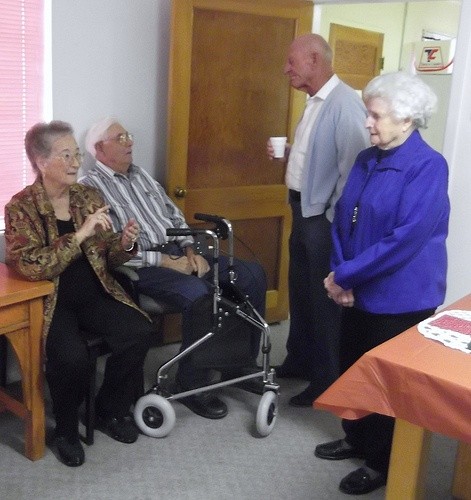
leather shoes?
[174,383,228,419]
[271,364,309,379]
[93,409,138,444]
[56,425,85,467]
[338,465,389,494]
[290,386,328,406]
[221,365,268,393]
[314,436,364,460]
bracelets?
[123,242,134,252]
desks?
[312,293,471,500]
[0,260,56,462]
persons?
[312,71,451,495]
[266,33,375,407]
[4,120,151,468]
[77,116,278,420]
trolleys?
[133,213,280,438]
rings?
[131,236,137,242]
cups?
[270,137,287,159]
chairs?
[83,335,108,446]
[134,292,188,400]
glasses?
[100,133,136,146]
[42,152,86,164]
